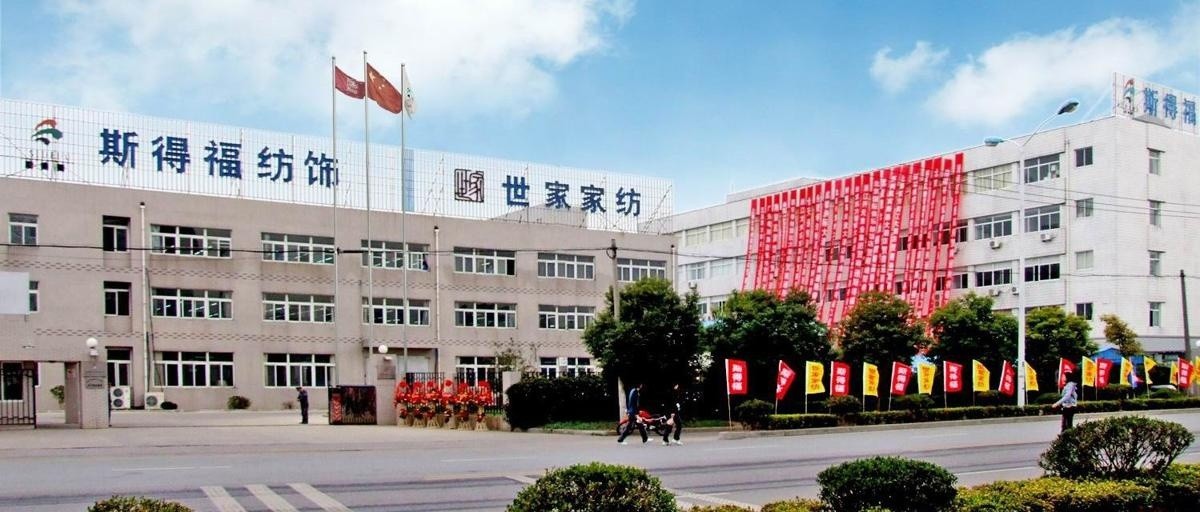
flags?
[367,63,402,114]
[403,67,416,119]
[335,66,365,99]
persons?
[1052,372,1078,433]
[296,386,309,424]
[617,381,653,444]
[662,383,683,446]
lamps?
[86,337,98,358]
[379,344,388,354]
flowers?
[438,379,458,406]
[393,380,412,408]
[426,379,442,401]
[410,382,427,404]
[473,381,496,407]
[451,383,474,404]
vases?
[411,403,426,427]
[457,401,473,430]
[443,404,457,429]
[427,400,439,428]
[397,402,410,426]
[474,405,489,431]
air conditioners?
[688,280,695,287]
[144,392,165,410]
[934,292,941,308]
[989,240,1000,248]
[1039,233,1052,241]
[109,386,131,409]
[1011,286,1019,294]
[917,301,923,309]
[988,288,998,296]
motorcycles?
[615,407,673,438]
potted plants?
[50,385,65,409]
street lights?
[983,98,1081,410]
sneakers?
[617,438,684,447]
